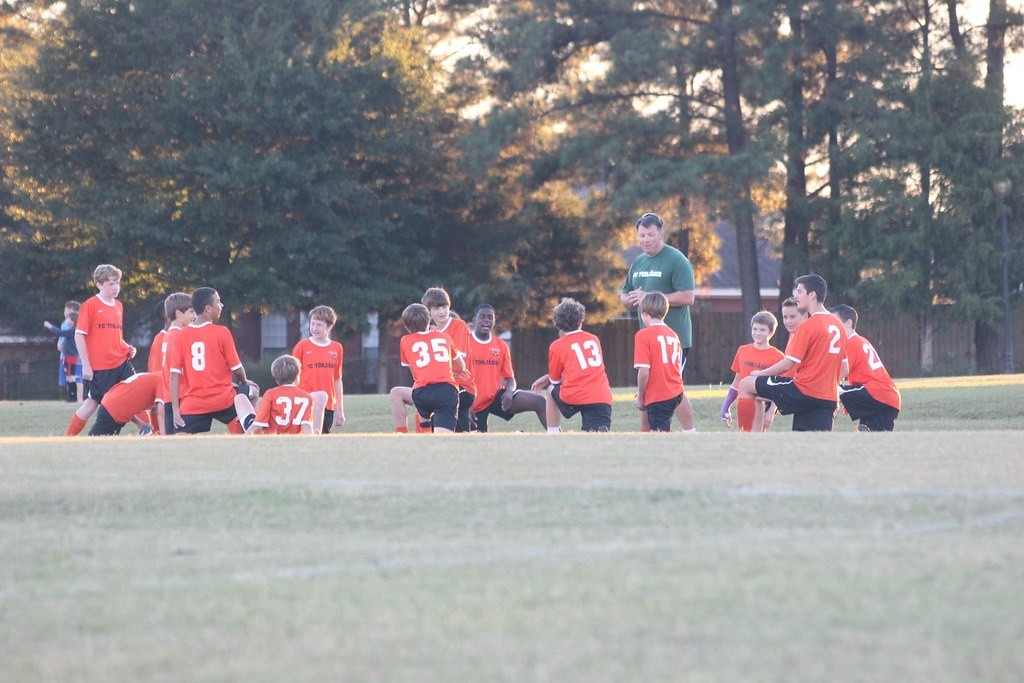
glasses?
[641,213,661,221]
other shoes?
[598,426,608,433]
[138,424,154,436]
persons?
[44,264,346,436]
[387,287,613,432]
[621,213,696,432]
[721,274,900,432]
